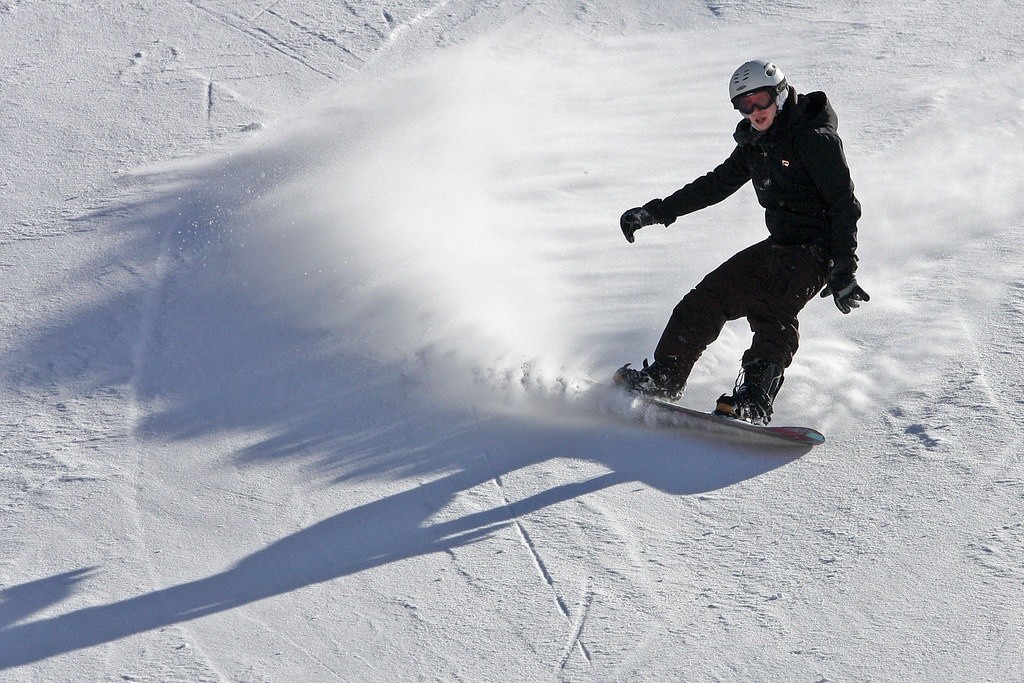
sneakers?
[716,382,773,427]
[613,358,686,403]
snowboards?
[581,377,827,447]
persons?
[614,60,869,428]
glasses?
[734,87,777,115]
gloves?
[820,249,870,316]
[619,198,667,243]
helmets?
[728,59,790,111]
